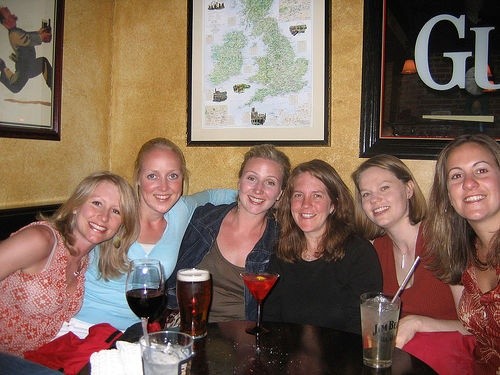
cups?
[139,330,195,375]
[175,267,211,339]
[359,291,401,369]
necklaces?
[73,272,79,276]
[401,253,404,269]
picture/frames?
[186,0,333,148]
[0,0,65,142]
[358,0,500,160]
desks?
[186,320,438,375]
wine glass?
[125,259,166,338]
[240,271,280,336]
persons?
[0,171,142,375]
[354,153,477,375]
[164,145,290,322]
[261,159,383,320]
[421,134,500,375]
[74,137,280,331]
